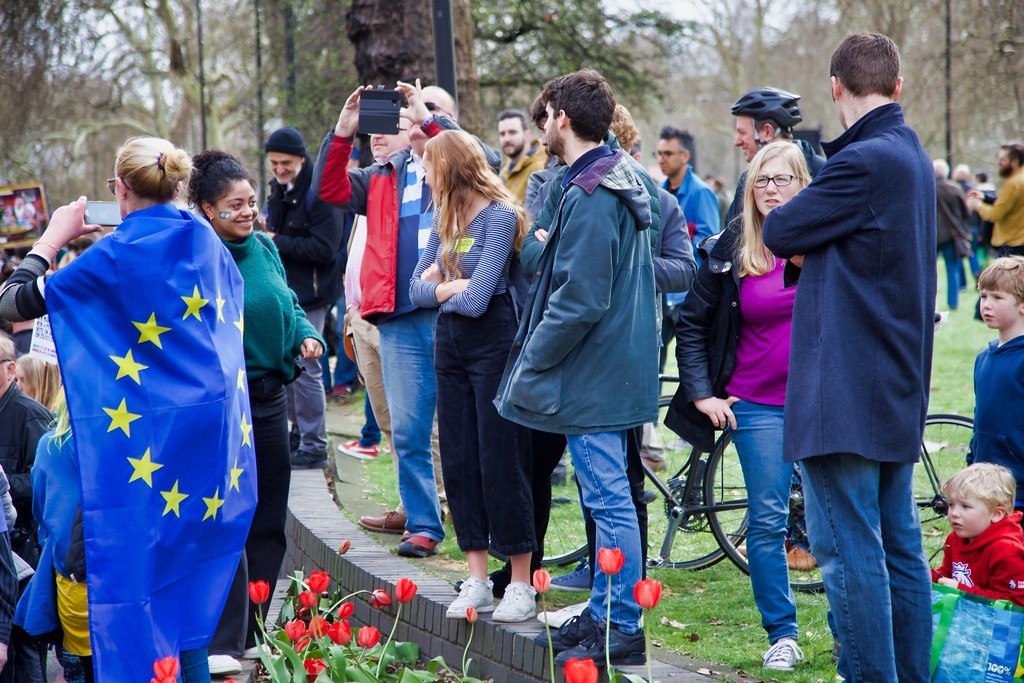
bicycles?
[490,377,975,594]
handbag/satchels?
[930,581,1024,683]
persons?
[0,33,1024,683]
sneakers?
[446,576,494,619]
[338,441,379,460]
[762,636,804,672]
[491,582,537,623]
[534,607,599,650]
[537,598,591,629]
[553,622,647,666]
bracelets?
[33,241,60,254]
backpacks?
[305,185,354,273]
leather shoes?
[358,510,407,533]
[738,539,793,559]
[784,546,818,570]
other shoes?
[290,450,328,470]
[208,654,243,675]
[326,385,352,403]
[243,644,272,658]
[454,560,541,601]
[550,555,591,592]
[398,535,438,557]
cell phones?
[84,201,122,226]
[358,90,401,135]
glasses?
[652,150,684,157]
[405,102,453,118]
[0,357,12,364]
[754,174,802,188]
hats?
[265,127,308,155]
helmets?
[731,87,802,127]
